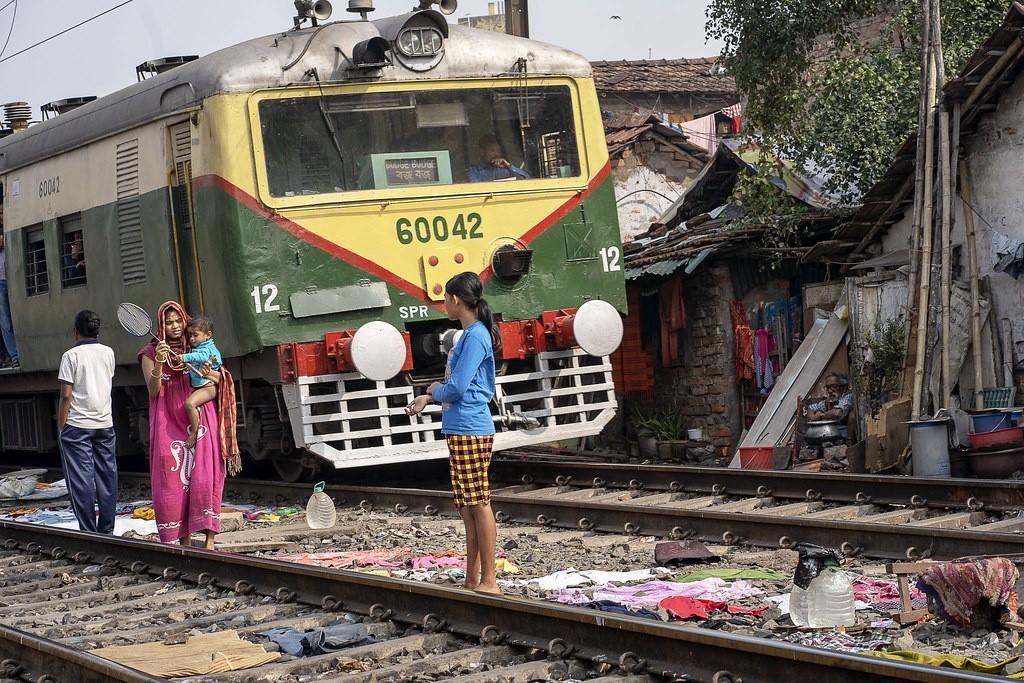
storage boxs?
[738,445,791,470]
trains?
[0,0,629,565]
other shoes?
[11,360,19,368]
[2,360,12,368]
[0,359,5,367]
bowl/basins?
[966,427,1024,449]
[0,469,48,483]
[967,446,1024,478]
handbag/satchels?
[656,539,720,568]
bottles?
[808,561,856,628]
[306,481,336,529]
[789,584,809,629]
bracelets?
[155,355,167,362]
[151,370,162,378]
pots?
[804,419,842,446]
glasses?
[823,384,841,391]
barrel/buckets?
[910,420,951,478]
[739,446,773,470]
[950,457,967,477]
[1011,410,1023,428]
[687,429,702,439]
[972,412,1011,433]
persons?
[0,194,20,368]
[464,135,531,182]
[58,309,115,534]
[174,314,222,448]
[137,301,242,551]
[404,271,503,594]
[65,232,87,286]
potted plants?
[629,404,704,458]
